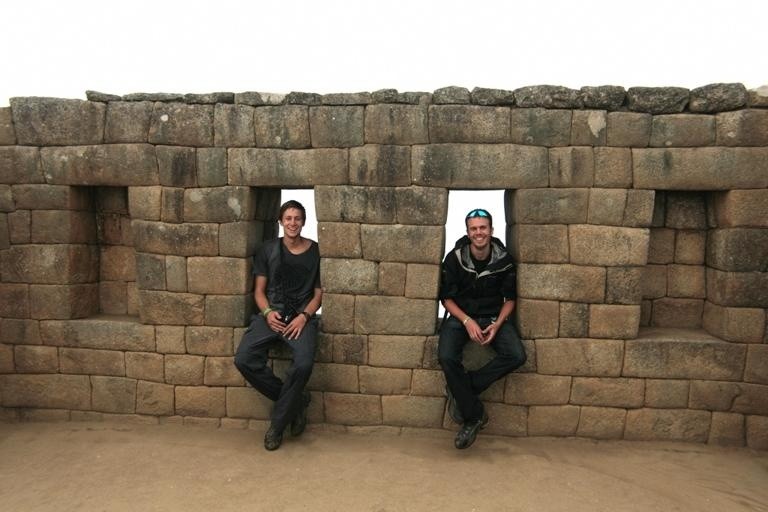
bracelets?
[462,316,472,326]
[260,306,276,320]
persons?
[232,200,322,450]
[438,209,527,450]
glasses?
[466,210,489,217]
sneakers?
[445,385,466,424]
[265,426,283,450]
[455,410,489,449]
[291,389,311,437]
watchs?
[301,309,311,323]
